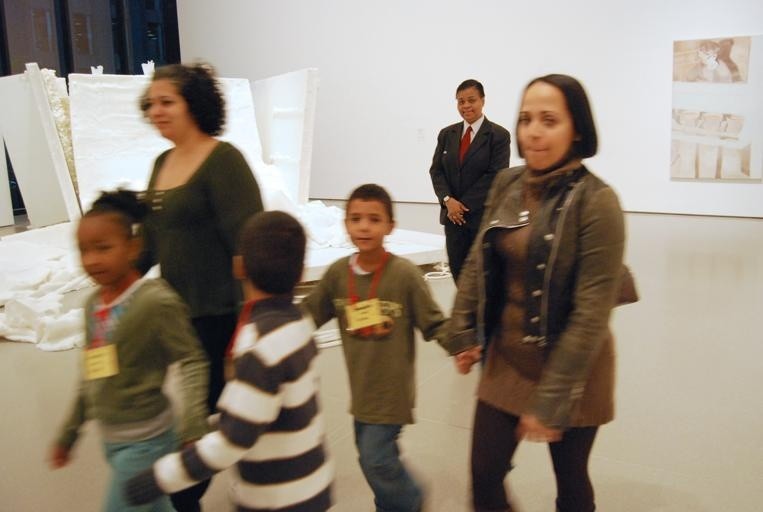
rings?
[453,216,456,218]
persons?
[45,190,210,512]
[301,185,478,512]
[445,75,626,512]
[429,79,510,286]
[137,64,264,512]
[125,210,334,512]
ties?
[460,126,472,166]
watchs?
[443,195,450,201]
